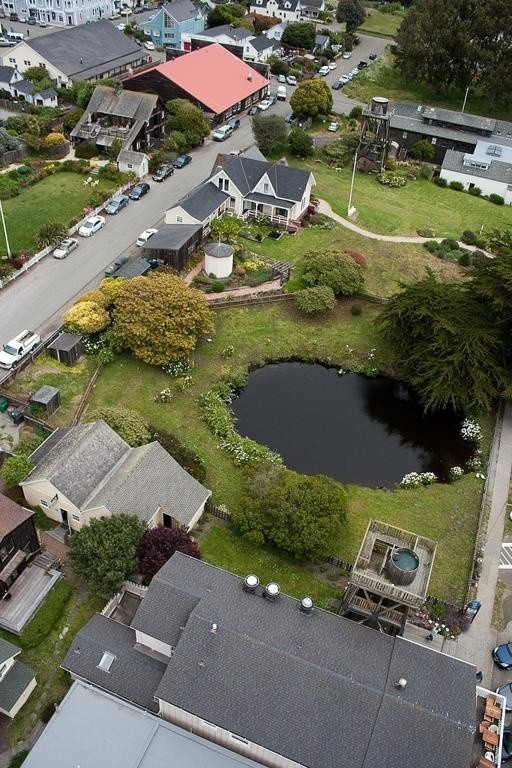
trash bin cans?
[106,272,113,279]
[11,409,24,424]
[144,256,157,269]
[0,400,9,412]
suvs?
[286,75,297,85]
[135,227,159,248]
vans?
[213,124,234,141]
[276,84,288,101]
[143,40,155,50]
[4,31,24,40]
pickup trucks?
[0,327,41,370]
[0,37,17,47]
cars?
[258,99,271,111]
[77,214,105,238]
[151,163,174,182]
[118,23,126,30]
[247,105,260,115]
[277,74,286,83]
[172,153,193,169]
[51,237,79,260]
[327,122,340,132]
[227,116,241,129]
[0,8,46,27]
[319,49,378,91]
[127,182,151,201]
[492,638,512,670]
[103,194,129,215]
[283,112,297,123]
[502,722,512,764]
[266,95,278,105]
[494,681,512,711]
[108,0,163,20]
